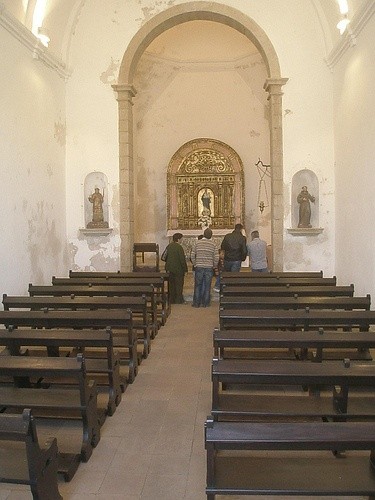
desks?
[134,243,159,270]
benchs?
[0,409,64,499]
[217,267,372,357]
[211,327,375,392]
[219,306,375,361]
[0,351,101,463]
[203,415,375,500]
[0,309,138,383]
[1,270,171,360]
[209,355,375,459]
[0,326,122,417]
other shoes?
[213,287,220,292]
[192,303,200,307]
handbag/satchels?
[160,248,168,261]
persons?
[165,233,188,303]
[246,231,268,272]
[212,249,224,293]
[201,189,212,216]
[190,235,204,304]
[297,185,315,227]
[88,188,104,224]
[221,224,248,272]
[191,228,219,307]
